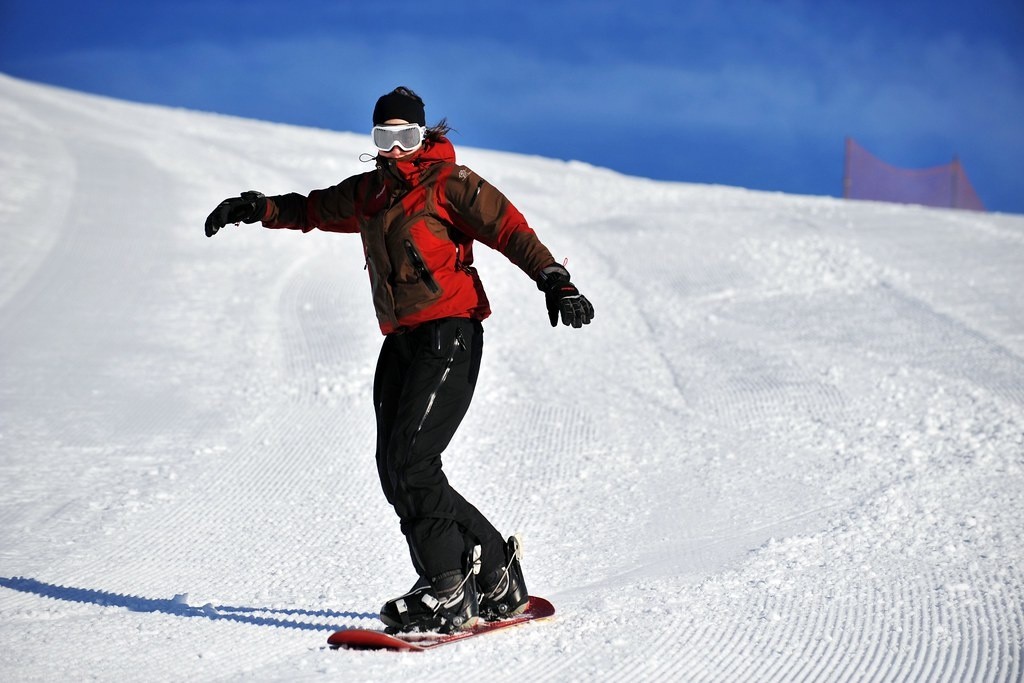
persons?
[204,85,597,635]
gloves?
[205,190,267,237]
[539,263,594,328]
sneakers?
[379,544,484,633]
[471,536,531,619]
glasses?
[372,123,426,151]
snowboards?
[326,595,555,650]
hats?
[372,92,426,128]
